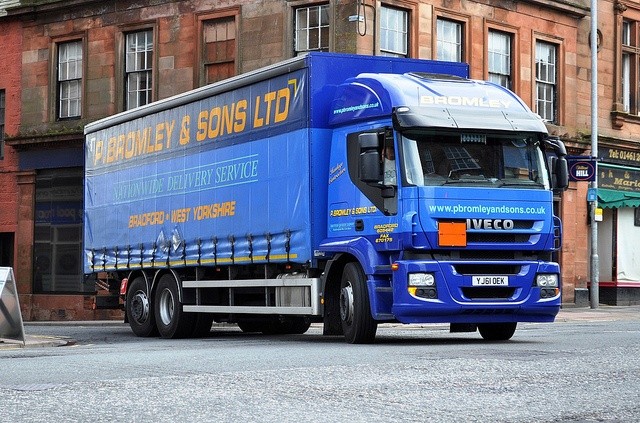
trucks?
[83,50,570,343]
